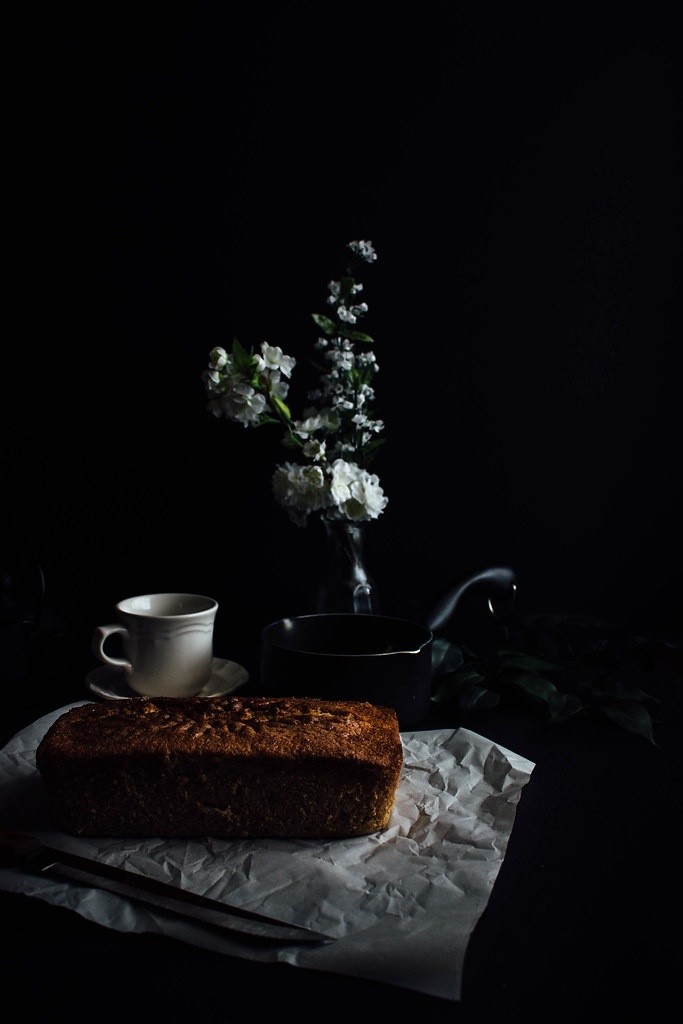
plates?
[88,658,248,699]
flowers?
[201,239,388,521]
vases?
[303,510,374,615]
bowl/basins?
[257,610,435,730]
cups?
[94,593,219,698]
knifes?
[0,829,337,942]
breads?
[35,698,403,838]
